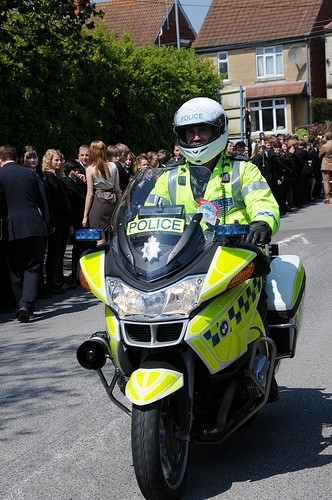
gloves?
[243,221,272,245]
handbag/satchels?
[75,223,102,243]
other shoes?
[53,280,76,289]
[17,307,29,322]
[263,370,280,404]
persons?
[106,107,332,217]
[81,139,122,246]
[133,97,280,404]
[0,144,50,322]
[20,144,91,298]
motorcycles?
[75,163,307,500]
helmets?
[172,97,228,165]
